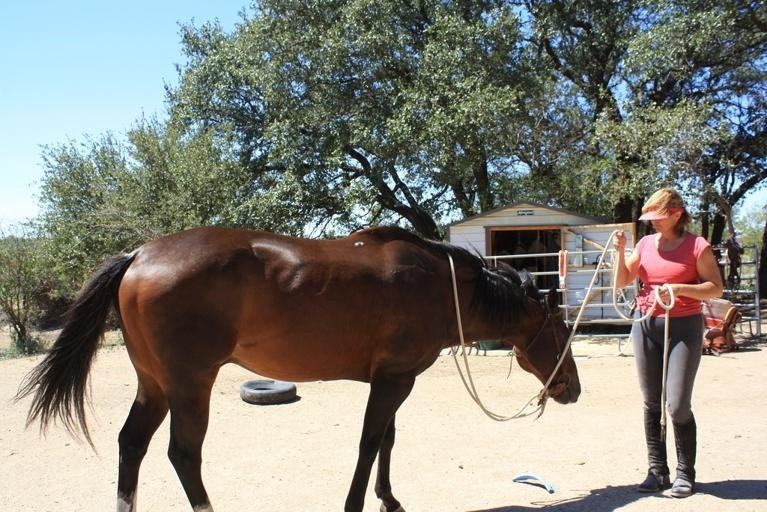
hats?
[636,203,685,222]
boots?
[637,409,672,493]
[672,412,697,498]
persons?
[720,230,743,287]
[609,185,726,501]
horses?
[9,225,581,511]
